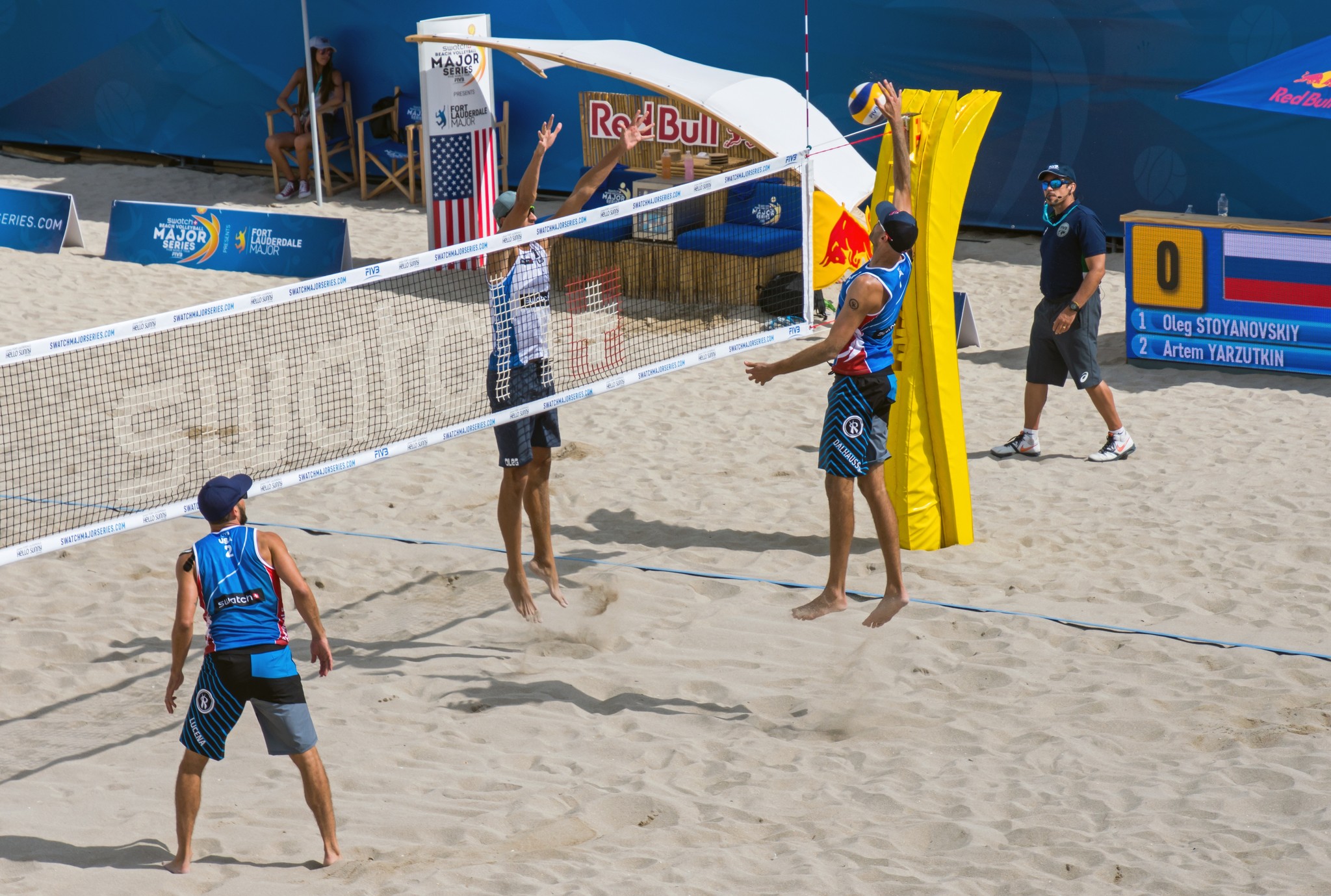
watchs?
[1068,298,1080,312]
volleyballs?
[848,82,891,126]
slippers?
[777,316,805,326]
[764,320,786,332]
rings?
[1063,326,1066,328]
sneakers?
[992,431,1041,458]
[298,180,312,199]
[275,181,299,201]
[1088,431,1136,462]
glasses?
[1042,179,1071,190]
[312,48,334,56]
[527,207,535,217]
[877,219,893,241]
[242,492,248,499]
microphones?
[1058,187,1072,199]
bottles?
[1218,193,1228,216]
[660,149,671,179]
[684,150,694,180]
[1184,205,1196,214]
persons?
[484,109,655,622]
[742,79,918,628]
[989,162,1136,462]
[163,473,344,873]
[266,34,343,200]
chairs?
[264,83,429,205]
[535,166,657,244]
[676,179,802,257]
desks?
[1116,207,1328,379]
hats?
[309,35,337,52]
[197,473,252,523]
[493,191,517,228]
[875,200,919,254]
[1038,162,1077,184]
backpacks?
[756,271,828,321]
[369,89,403,139]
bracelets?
[291,113,298,117]
